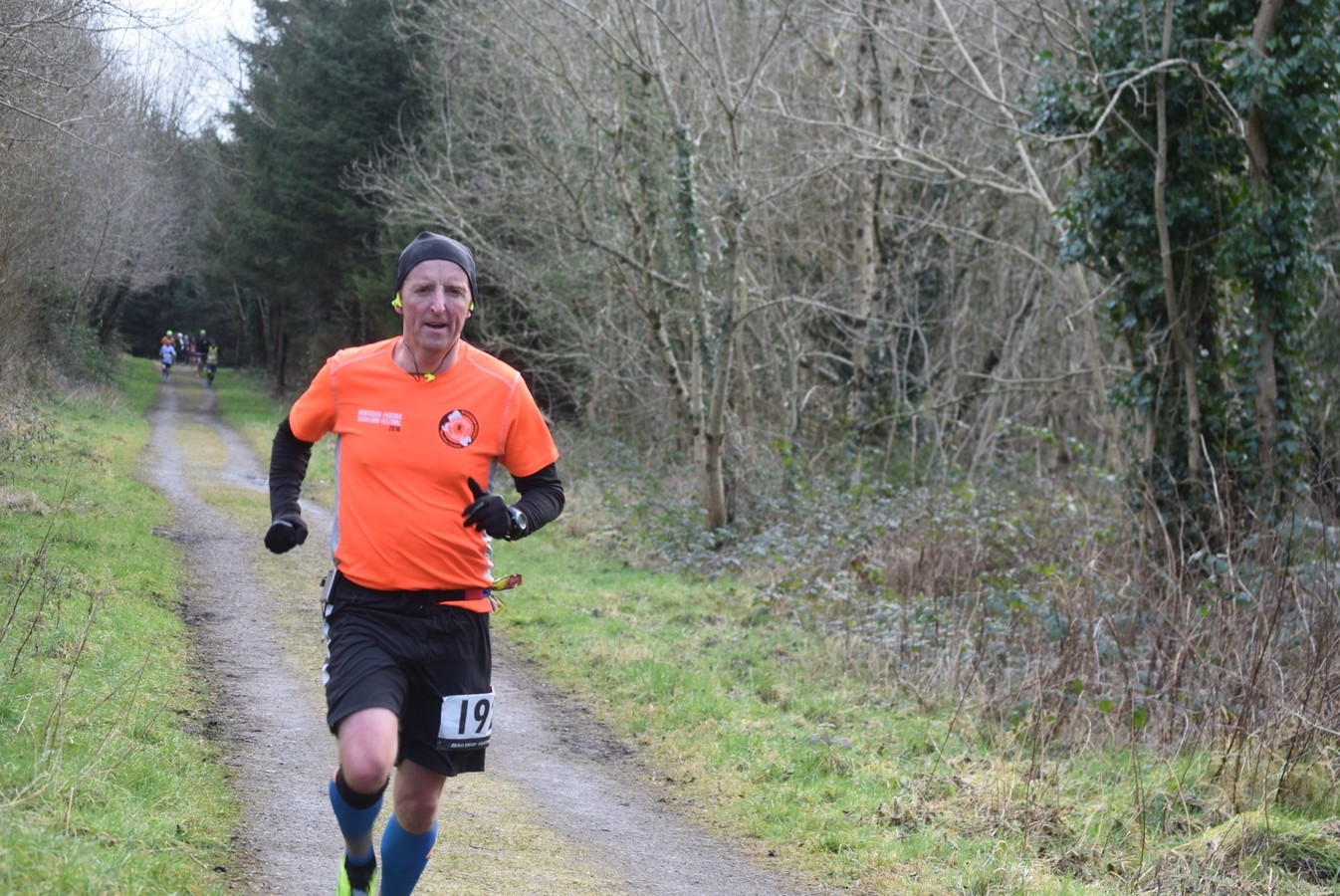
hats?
[200,330,206,335]
[166,330,173,335]
[394,231,478,304]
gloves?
[462,478,513,539]
[264,519,308,554]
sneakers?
[337,853,377,896]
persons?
[160,326,222,387]
[264,230,566,895]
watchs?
[507,504,529,540]
